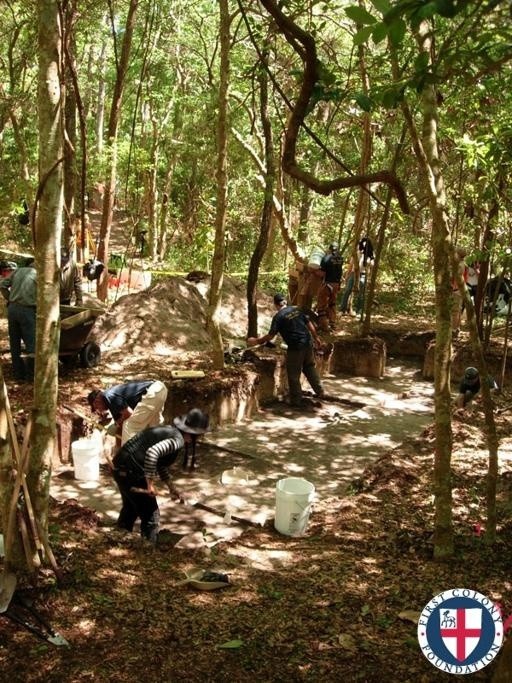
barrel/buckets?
[70,438,101,481]
[128,269,152,290]
[273,475,316,538]
[116,268,130,283]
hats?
[274,294,284,304]
[88,390,100,412]
[173,409,209,434]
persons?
[457,367,484,412]
[111,407,206,539]
[315,241,480,332]
[88,380,168,447]
[248,294,324,406]
[0,259,37,379]
[60,248,82,307]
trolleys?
[59,304,105,368]
